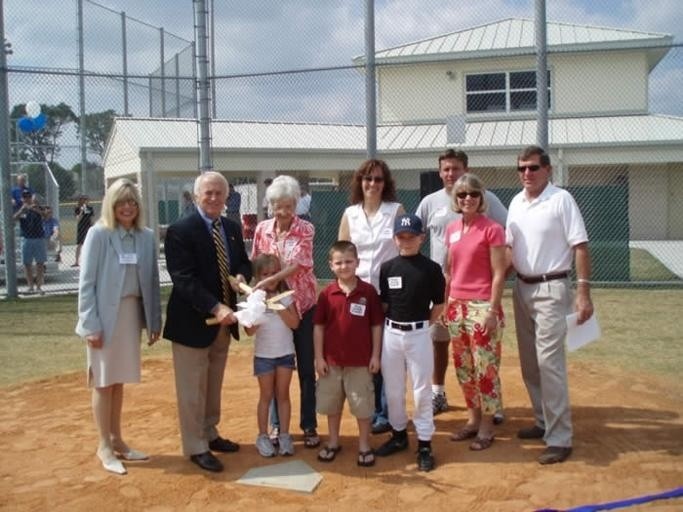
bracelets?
[488,308,500,316]
[576,277,589,285]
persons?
[40,206,61,262]
[234,178,258,240]
[415,148,509,425]
[376,214,447,472]
[249,176,321,448]
[226,185,242,230]
[12,174,35,236]
[75,178,163,474]
[232,254,300,458]
[263,178,272,221]
[439,173,505,450]
[295,184,312,222]
[13,190,49,293]
[312,240,384,466]
[504,147,595,465]
[180,191,195,220]
[163,172,253,473]
[72,192,94,267]
[337,159,408,433]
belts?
[386,320,423,331]
[517,272,567,285]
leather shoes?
[209,437,238,452]
[517,425,545,438]
[539,445,572,464]
[416,446,434,471]
[375,435,407,456]
[191,450,223,472]
[371,421,390,434]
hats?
[393,215,422,234]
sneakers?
[279,436,293,456]
[256,436,275,456]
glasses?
[363,175,384,182]
[456,190,480,198]
[518,165,545,172]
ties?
[210,220,231,307]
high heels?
[97,448,126,475]
[111,444,147,460]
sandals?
[470,431,495,450]
[269,427,280,445]
[451,425,477,440]
[303,430,320,448]
[432,393,448,415]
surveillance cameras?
[446,69,454,78]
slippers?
[317,444,341,462]
[358,449,375,466]
